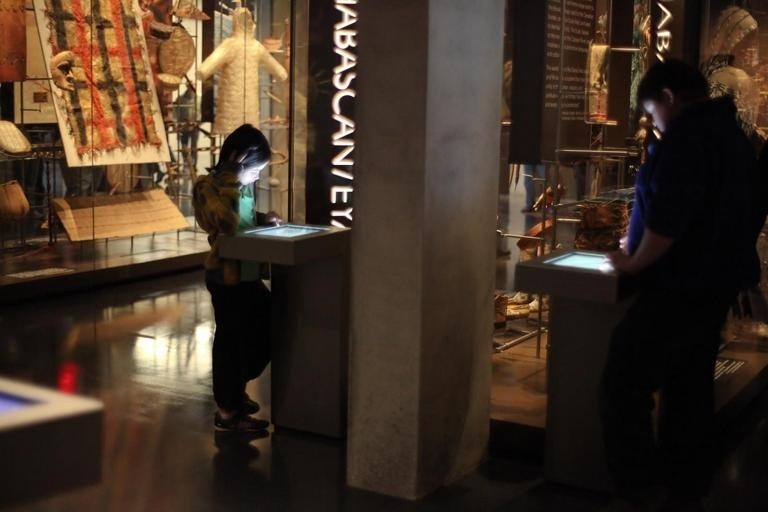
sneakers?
[215,430,269,464]
[214,392,269,432]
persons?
[597,58,761,511]
[191,122,282,433]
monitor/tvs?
[255,226,319,237]
[555,255,605,270]
